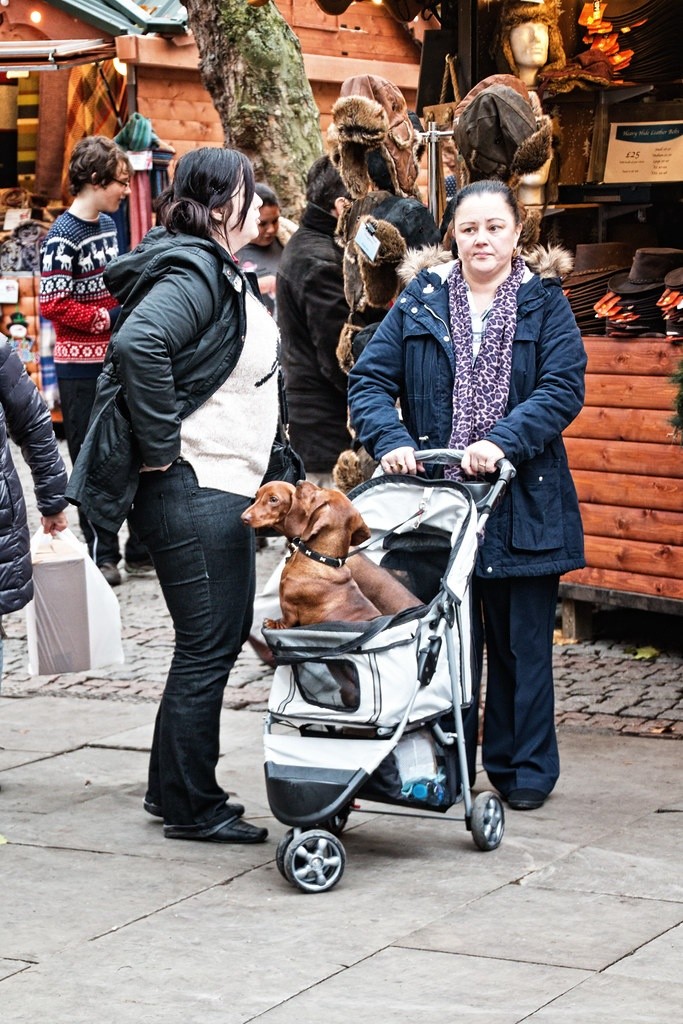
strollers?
[256,446,516,891]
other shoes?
[507,784,547,810]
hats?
[333,190,460,375]
[324,73,419,200]
[494,0,568,78]
[450,73,553,183]
[559,241,683,337]
[602,0,683,84]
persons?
[234,124,464,548]
[0,332,68,617]
[41,134,157,591]
[64,147,281,842]
[348,181,587,811]
[510,18,550,87]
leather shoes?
[141,797,269,845]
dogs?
[241,479,426,631]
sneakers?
[124,554,155,574]
[98,561,122,585]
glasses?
[108,175,130,190]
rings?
[390,465,397,472]
[397,463,405,470]
[479,462,485,468]
